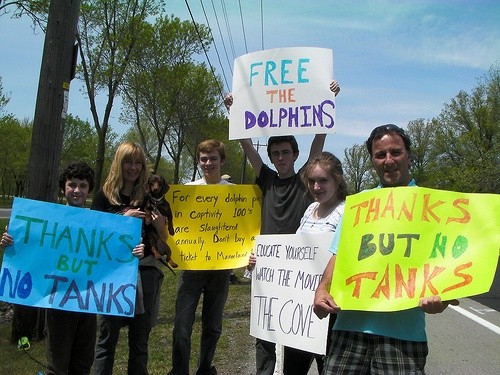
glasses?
[375,124,405,136]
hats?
[221,174,231,181]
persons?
[223,80,340,375]
[313,124,460,375]
[247,152,349,375]
[0,160,145,375]
[167,139,238,375]
[89,140,168,375]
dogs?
[143,175,179,278]
